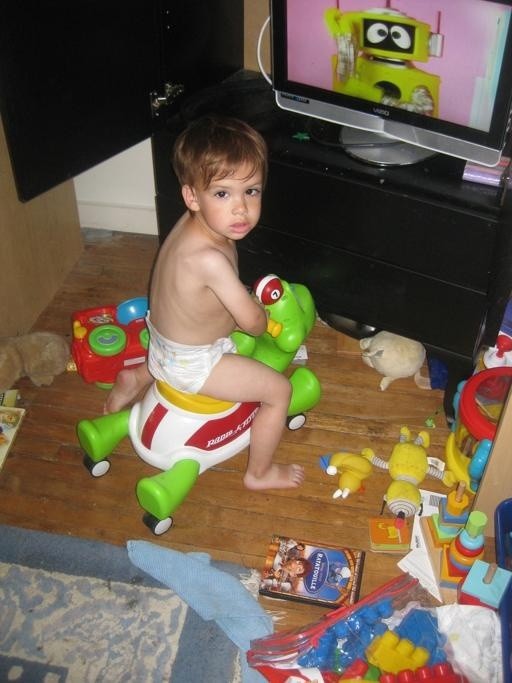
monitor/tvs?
[269,0,512,168]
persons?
[102,115,305,491]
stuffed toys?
[0,331,69,392]
[318,450,371,498]
[362,427,455,529]
[359,330,431,391]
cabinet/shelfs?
[153,68,512,373]
[74,135,161,235]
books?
[0,406,27,470]
[368,517,410,555]
[259,534,363,608]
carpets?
[0,524,259,683]
